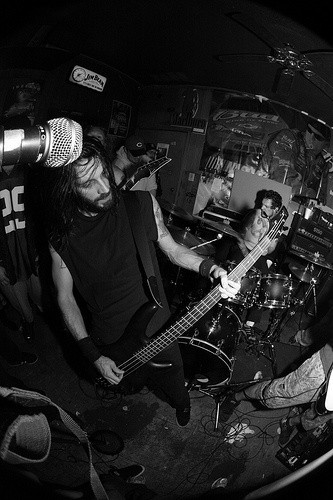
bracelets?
[199,259,215,276]
[78,337,101,362]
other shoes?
[110,463,145,482]
[218,391,241,424]
[279,417,295,443]
[175,407,191,428]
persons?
[0,385,155,500]
[47,135,242,427]
[112,136,157,198]
[226,189,288,337]
[254,120,333,198]
[217,337,333,447]
[0,116,52,340]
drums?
[217,258,263,309]
[178,302,243,390]
[255,272,294,310]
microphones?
[0,117,84,169]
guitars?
[59,206,287,390]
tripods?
[245,265,321,363]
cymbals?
[161,204,193,221]
[166,225,216,255]
[289,261,320,284]
[290,248,333,270]
[195,215,241,239]
